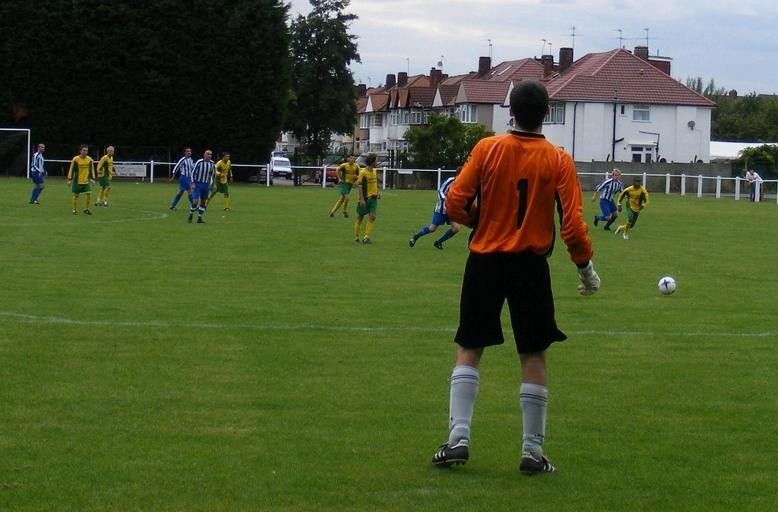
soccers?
[658,277,675,295]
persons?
[27,143,115,215]
[167,142,234,224]
[409,166,462,249]
[746,167,764,202]
[354,153,381,244]
[592,168,624,231]
[329,153,360,218]
[614,175,649,241]
[432,78,601,474]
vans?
[355,151,388,185]
[270,157,292,179]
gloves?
[576,259,601,296]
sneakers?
[432,437,469,464]
[594,215,599,226]
[434,241,443,249]
[409,238,416,248]
[84,210,92,215]
[604,225,611,231]
[363,238,371,244]
[72,209,80,216]
[519,453,555,475]
[623,233,628,239]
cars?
[315,163,341,184]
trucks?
[270,151,288,159]
